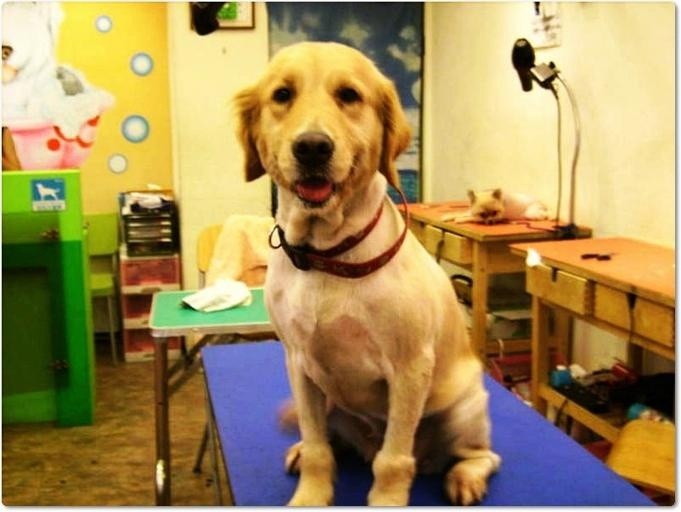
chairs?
[82,210,121,365]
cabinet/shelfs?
[119,190,181,361]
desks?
[193,339,659,506]
[149,290,279,506]
[397,199,594,384]
[505,235,676,443]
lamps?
[509,40,567,234]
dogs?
[440,187,548,224]
[229,40,503,507]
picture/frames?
[188,3,256,30]
